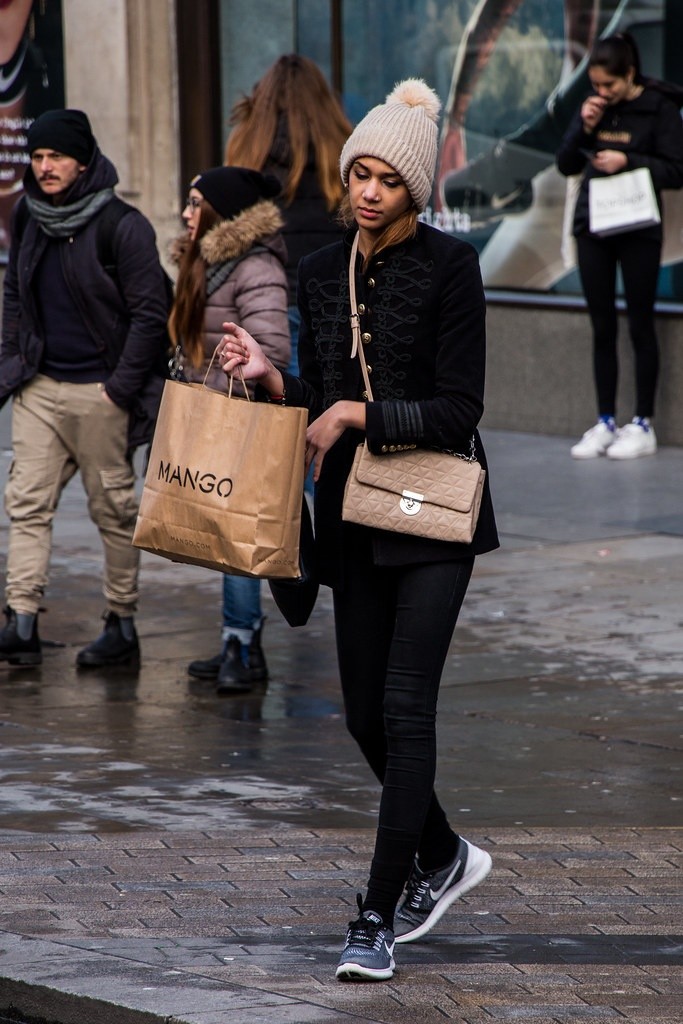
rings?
[221,351,225,357]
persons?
[160,167,291,685]
[0,110,162,667]
[224,54,359,502]
[0,0,65,266]
[555,34,683,461]
[217,79,501,985]
[435,0,683,292]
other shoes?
[217,615,268,691]
[188,653,220,679]
[1,604,49,666]
[75,609,140,667]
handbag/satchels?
[340,438,486,544]
[588,168,661,237]
[130,341,309,580]
[268,493,321,627]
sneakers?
[394,833,493,943]
[570,422,619,458]
[607,422,658,459]
[335,892,396,980]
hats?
[340,77,442,214]
[190,166,283,219]
[26,108,97,166]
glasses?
[185,198,205,216]
